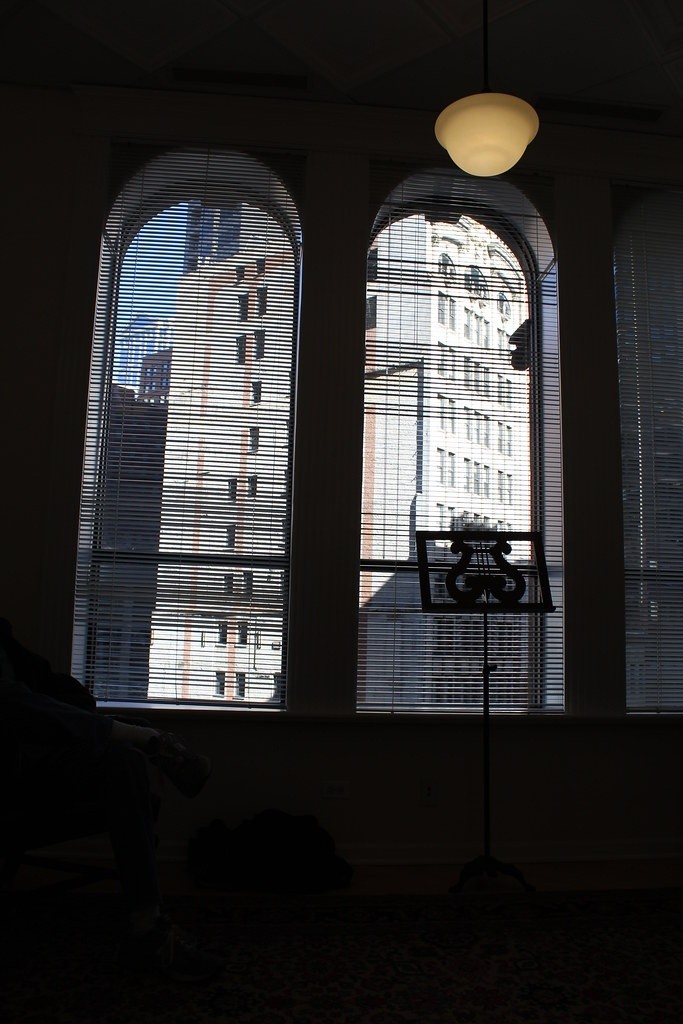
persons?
[1,616,216,981]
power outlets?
[322,779,351,800]
[418,779,439,806]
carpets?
[0,886,683,1024]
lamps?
[434,0,540,177]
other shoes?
[128,922,225,983]
[144,729,215,801]
